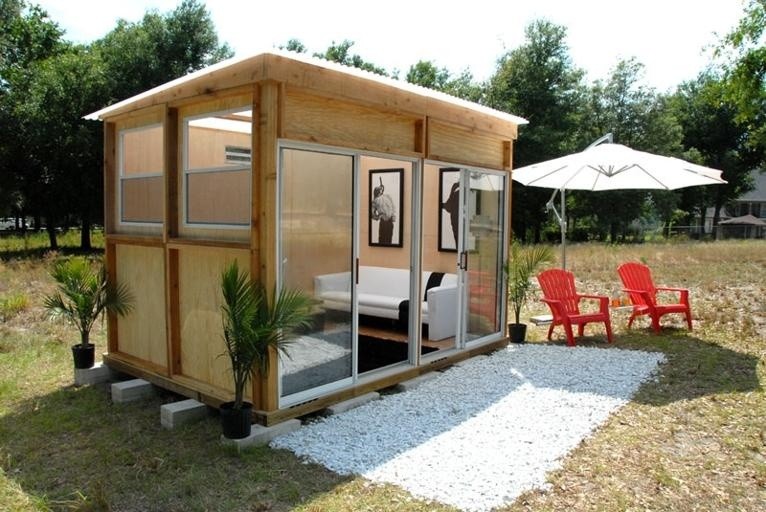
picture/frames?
[438,168,478,254]
[367,168,403,249]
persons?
[371,186,398,245]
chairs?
[537,269,612,347]
[617,262,692,334]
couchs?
[313,266,459,342]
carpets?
[279,332,437,398]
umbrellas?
[511,142,731,271]
[717,213,766,240]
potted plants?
[217,257,316,438]
[507,241,558,343]
[40,253,137,370]
